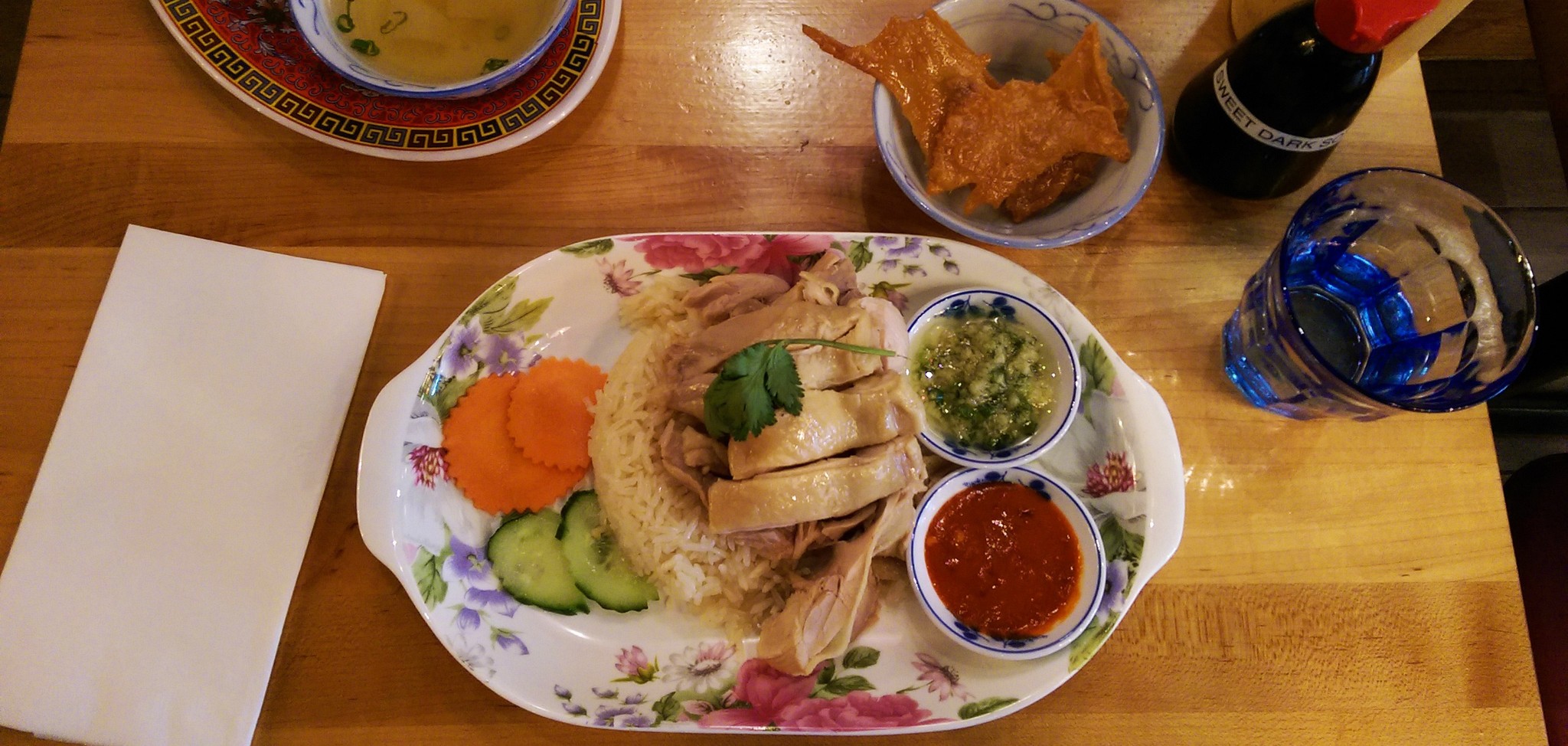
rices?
[585,275,810,640]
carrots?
[442,372,585,516]
[507,356,608,473]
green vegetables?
[915,325,1040,451]
[702,338,896,442]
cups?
[904,464,1108,659]
[1233,1,1469,86]
[902,288,1083,470]
[1222,165,1543,424]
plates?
[355,231,1185,737]
[146,0,622,164]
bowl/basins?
[288,0,577,97]
[872,0,1167,247]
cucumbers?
[556,487,660,613]
[487,508,591,616]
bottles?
[1166,0,1440,202]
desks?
[0,0,1555,746]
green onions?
[335,13,508,71]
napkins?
[0,223,391,746]
